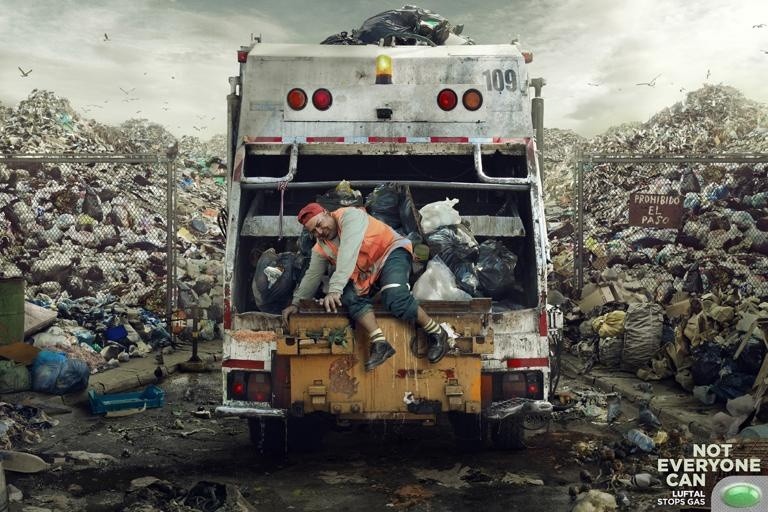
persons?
[278,204,451,370]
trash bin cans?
[0,276,25,346]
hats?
[297,202,325,225]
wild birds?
[607,395,623,424]
[706,69,711,78]
[118,86,141,103]
[102,32,110,42]
[192,113,219,131]
[632,382,654,394]
[618,472,662,492]
[752,23,767,29]
[637,401,661,428]
[634,72,663,87]
[80,104,105,113]
[17,66,34,77]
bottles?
[629,429,659,454]
[414,244,429,262]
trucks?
[216,32,562,449]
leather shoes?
[428,326,449,364]
[364,340,395,372]
